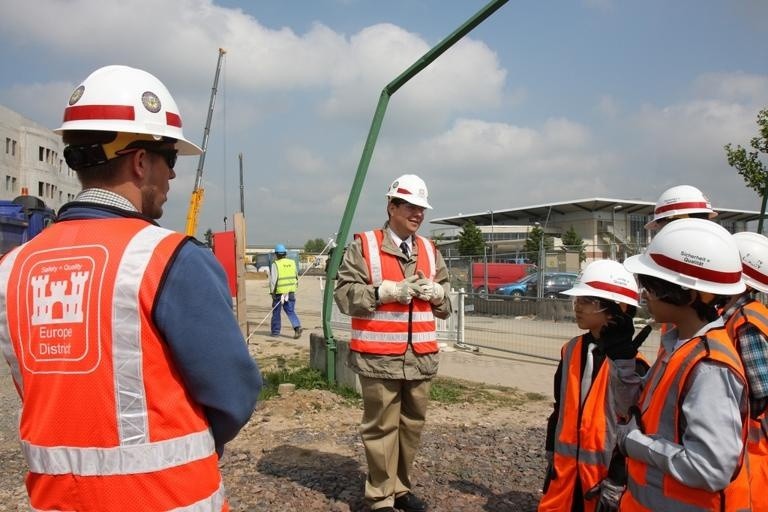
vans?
[467,260,541,300]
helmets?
[557,259,643,311]
[274,243,287,254]
[622,216,747,296]
[730,231,768,295]
[50,64,206,157]
[384,174,434,211]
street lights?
[612,204,623,243]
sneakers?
[293,327,304,339]
[270,333,279,337]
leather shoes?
[374,506,398,512]
[393,491,429,512]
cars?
[494,268,577,303]
[526,274,579,302]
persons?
[269,244,302,339]
[722,231,768,512]
[334,174,453,512]
[1,65,261,512]
[600,217,754,511]
[536,260,651,511]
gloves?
[614,405,646,458]
[600,313,652,359]
[583,477,627,512]
[377,273,424,306]
[413,269,446,307]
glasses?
[643,284,672,302]
[572,297,609,311]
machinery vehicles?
[186,47,228,240]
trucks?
[0,195,59,263]
